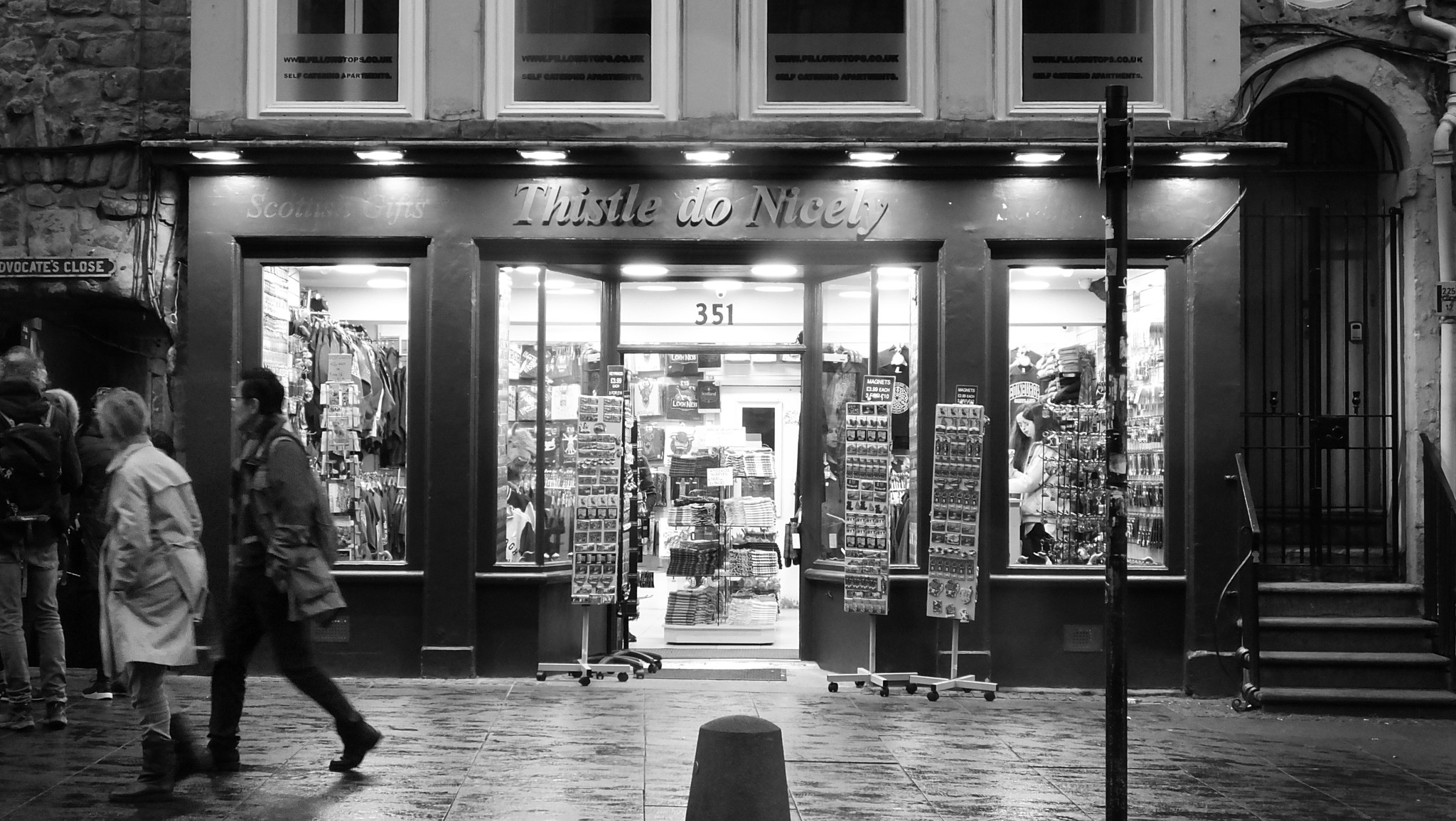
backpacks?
[246,434,337,567]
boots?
[170,712,215,781]
[109,736,174,800]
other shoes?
[193,754,241,772]
[329,720,381,772]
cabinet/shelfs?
[1037,398,1106,565]
[662,446,779,643]
[1126,383,1163,547]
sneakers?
[82,682,113,700]
[1,686,44,701]
[36,703,67,725]
[0,703,35,728]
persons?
[195,366,383,771]
[95,387,204,802]
[1009,403,1069,565]
[0,346,132,731]
[622,453,658,642]
